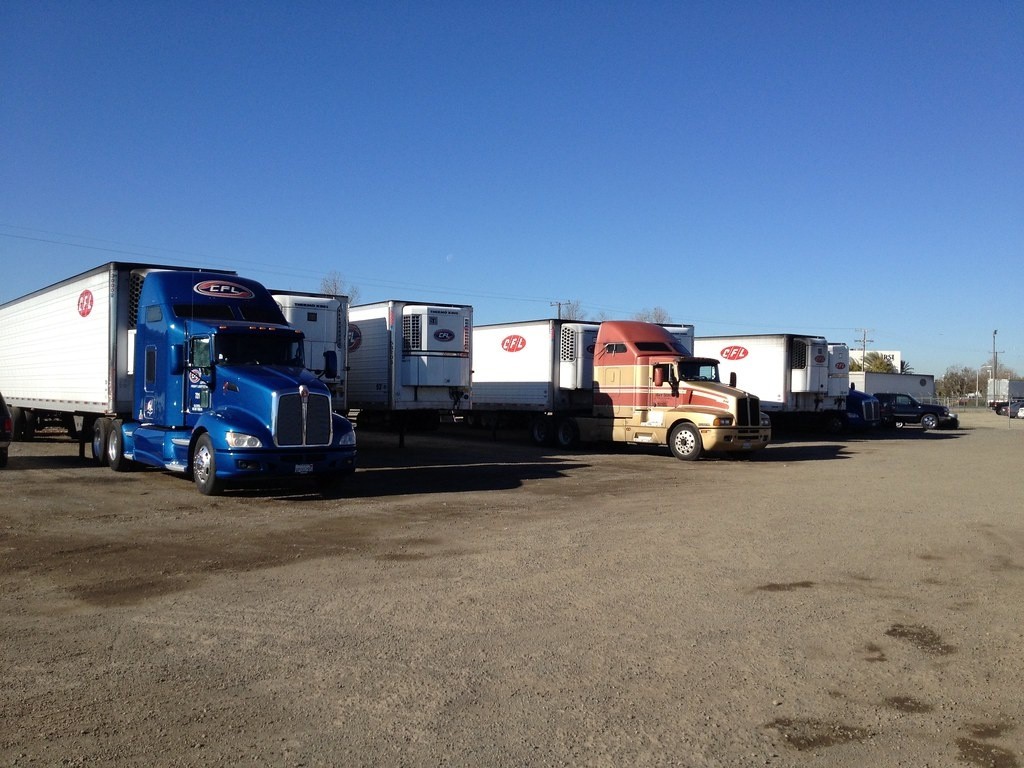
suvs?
[871,392,950,430]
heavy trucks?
[458,317,772,460]
[0,259,360,498]
